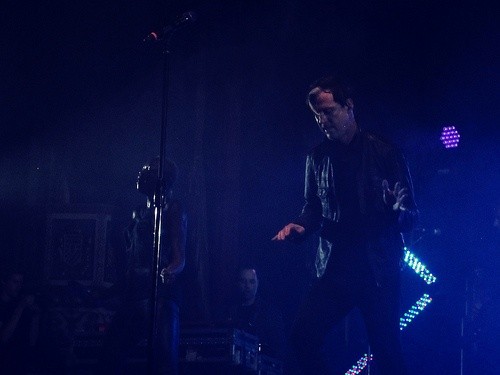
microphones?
[142,10,197,45]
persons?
[226,256,275,375]
[272,81,417,375]
[113,158,187,375]
